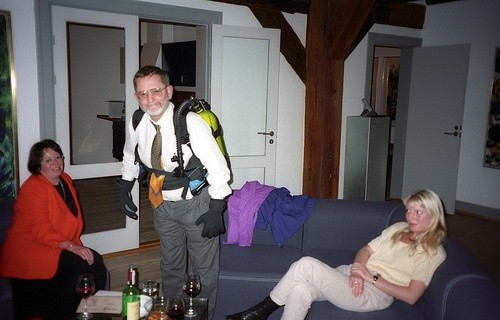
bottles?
[146,297,170,320]
[122,263,141,320]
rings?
[354,282,358,284]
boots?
[226,296,280,320]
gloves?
[195,199,227,238]
[119,178,138,220]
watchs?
[371,273,381,285]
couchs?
[213,199,500,320]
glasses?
[41,154,64,164]
[137,84,168,100]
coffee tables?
[92,297,209,320]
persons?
[120,66,232,320]
[0,139,107,320]
[224,190,447,320]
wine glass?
[76,274,93,320]
[182,274,202,318]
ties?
[148,119,165,209]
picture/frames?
[482,45,500,171]
[378,56,401,127]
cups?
[139,280,160,300]
[167,295,187,320]
